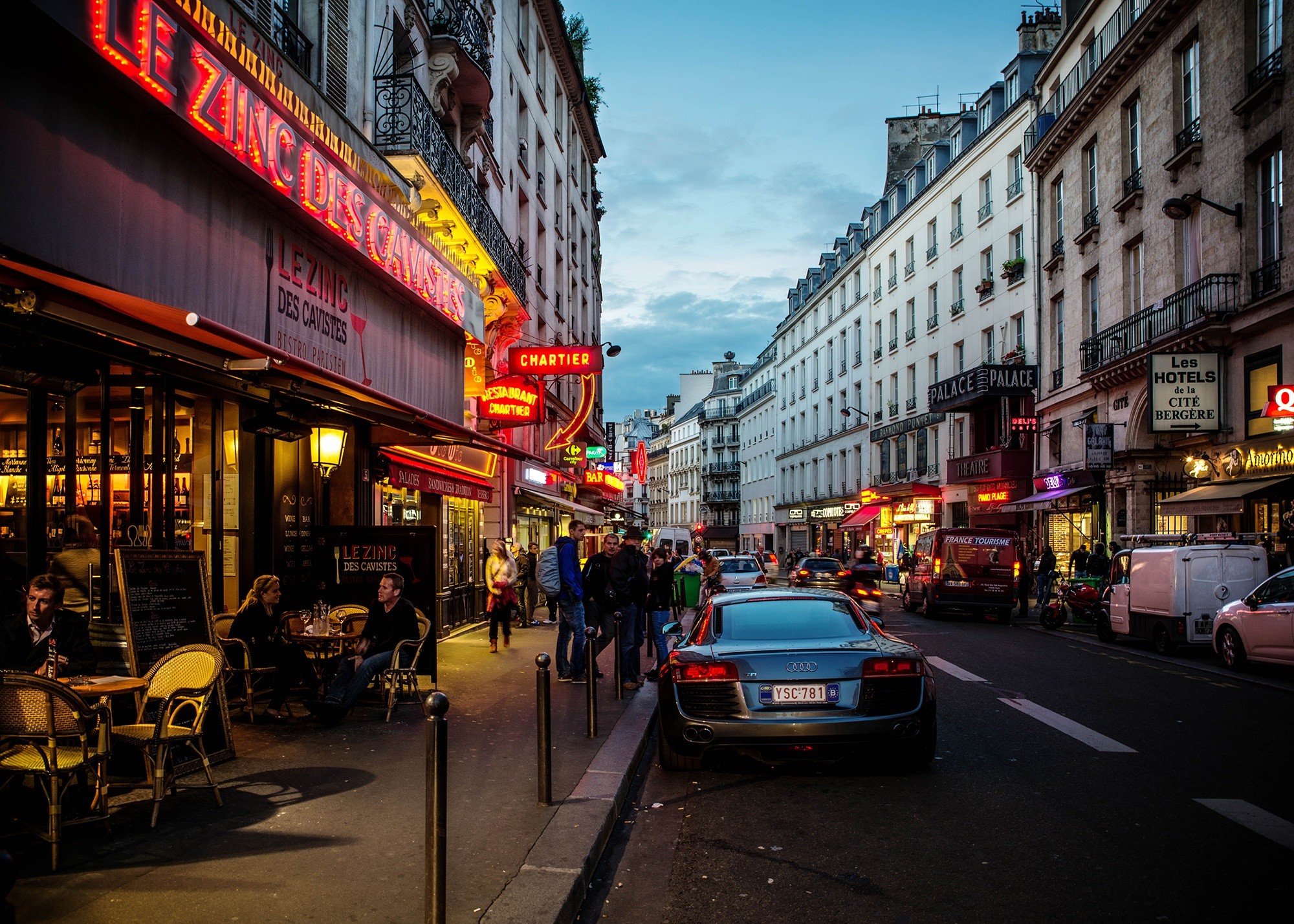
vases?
[974,277,991,293]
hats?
[621,526,647,541]
[697,550,711,558]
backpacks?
[536,540,578,598]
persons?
[299,572,421,729]
[586,526,650,689]
[521,542,541,625]
[508,542,526,629]
[550,520,598,684]
[542,592,559,625]
[642,534,1126,611]
[49,515,103,626]
[1258,542,1277,578]
[643,548,674,683]
[3,574,100,681]
[228,575,326,719]
[578,533,625,677]
[486,538,517,652]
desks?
[39,671,150,841]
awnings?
[1000,483,1097,540]
[839,502,890,533]
[0,255,649,525]
[1156,476,1292,535]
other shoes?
[644,668,660,680]
[531,620,541,626]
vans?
[651,527,693,564]
[902,527,1020,620]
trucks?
[1097,531,1273,650]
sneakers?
[504,635,510,648]
[622,681,641,690]
[489,638,497,653]
[571,671,598,683]
[558,674,572,682]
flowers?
[1002,343,1024,365]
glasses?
[629,537,644,543]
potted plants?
[1001,255,1025,278]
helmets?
[854,544,872,559]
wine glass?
[335,609,346,636]
[300,610,310,636]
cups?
[312,603,330,636]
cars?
[705,549,729,558]
[1212,565,1294,668]
[737,551,779,584]
[786,557,848,594]
[717,555,768,593]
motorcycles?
[845,564,883,618]
[1039,569,1101,630]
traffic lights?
[697,548,700,551]
[697,525,701,534]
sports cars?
[655,587,937,770]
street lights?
[306,421,349,525]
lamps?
[305,405,349,485]
[840,406,869,421]
[600,341,621,357]
[1162,193,1243,228]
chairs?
[1,669,111,871]
[93,641,229,828]
[213,605,432,723]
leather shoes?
[593,668,603,678]
[544,618,549,621]
[515,622,527,629]
[543,619,557,624]
